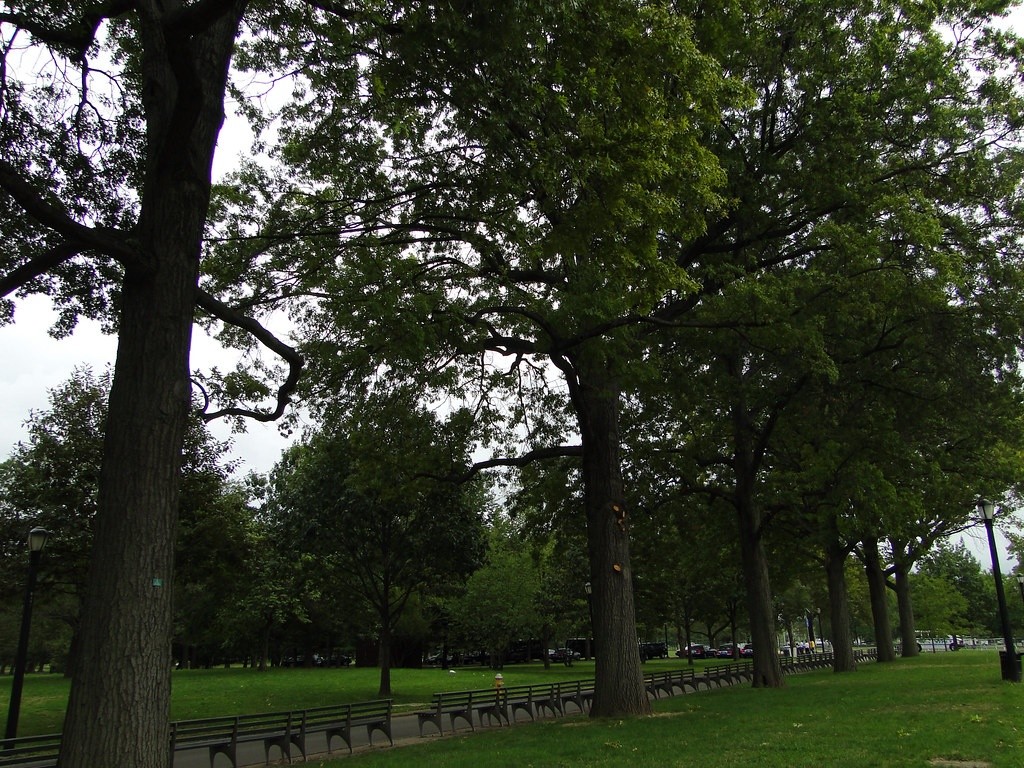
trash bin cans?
[998,650,1024,684]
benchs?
[695,661,753,691]
[413,668,697,737]
[0,698,393,768]
[853,648,877,663]
[779,652,834,676]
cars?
[639,642,755,659]
[551,648,581,663]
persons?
[782,639,815,657]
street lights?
[585,582,593,635]
[816,606,826,654]
[5,519,48,749]
[978,497,1021,682]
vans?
[914,629,964,651]
